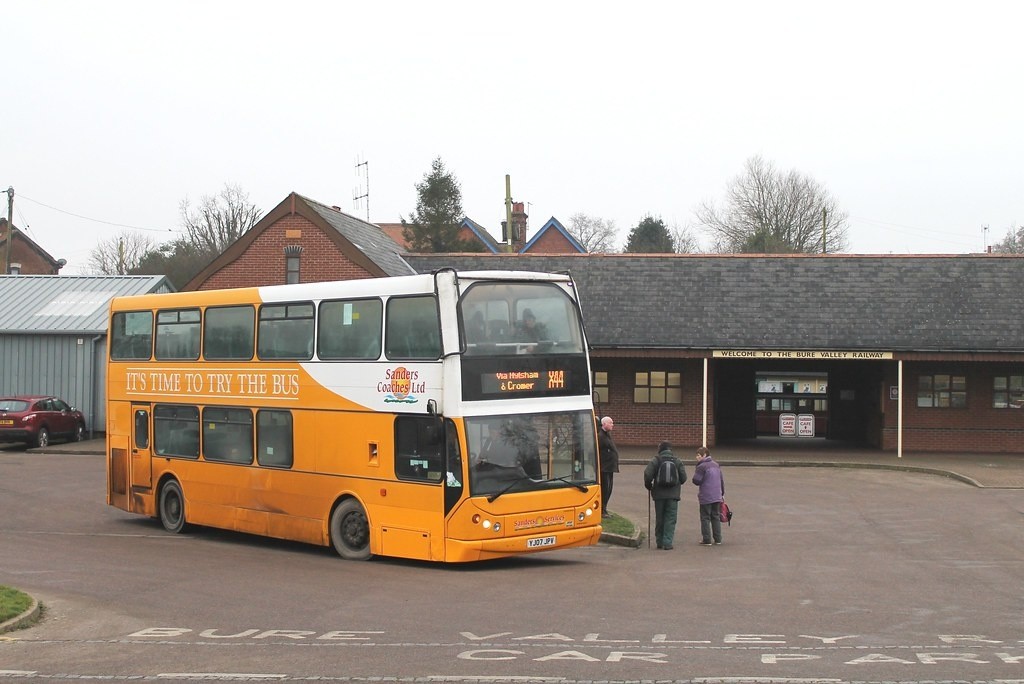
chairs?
[125,316,441,358]
[490,319,510,343]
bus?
[104,267,604,566]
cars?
[0,394,86,448]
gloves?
[645,481,652,490]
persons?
[597,416,619,518]
[692,447,725,545]
[644,440,687,550]
[480,422,518,466]
[469,308,552,342]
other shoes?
[699,541,712,546]
[602,512,609,518]
[715,541,721,544]
[606,511,613,517]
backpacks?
[654,456,682,488]
[720,496,732,522]
